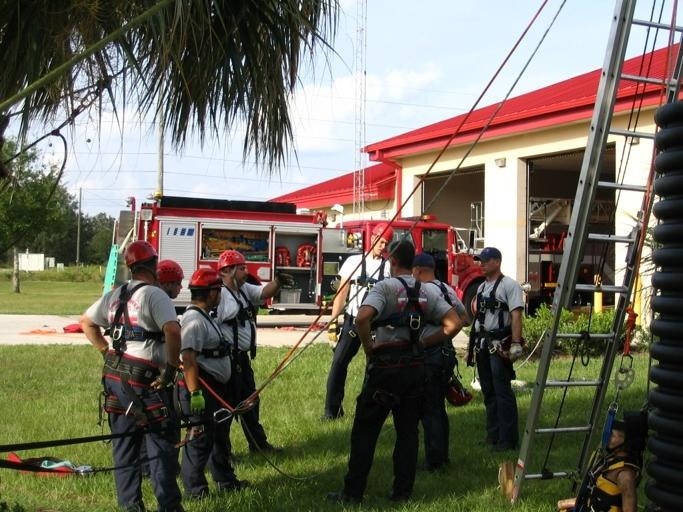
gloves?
[510,343,523,359]
[190,389,208,414]
[326,322,340,342]
[275,272,295,286]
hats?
[472,246,504,263]
[413,250,435,269]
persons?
[209,250,295,457]
[79,241,185,512]
[558,419,638,512]
[465,248,525,452]
[178,268,252,501]
[140,259,184,478]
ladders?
[510,0,683,504]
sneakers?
[216,479,251,492]
[325,490,360,505]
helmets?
[449,376,472,407]
[216,250,246,268]
[372,220,395,241]
[122,239,185,283]
[189,268,223,288]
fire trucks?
[469,198,616,308]
[110,194,485,328]
